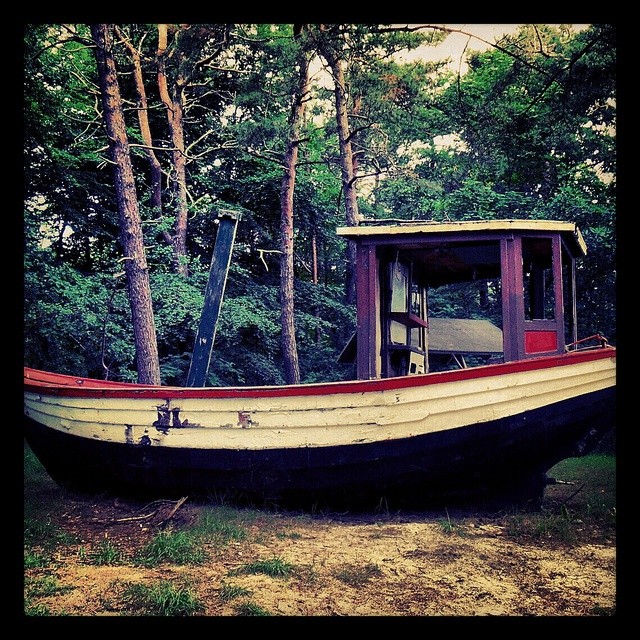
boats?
[25,218,616,513]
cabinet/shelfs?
[382,261,428,377]
